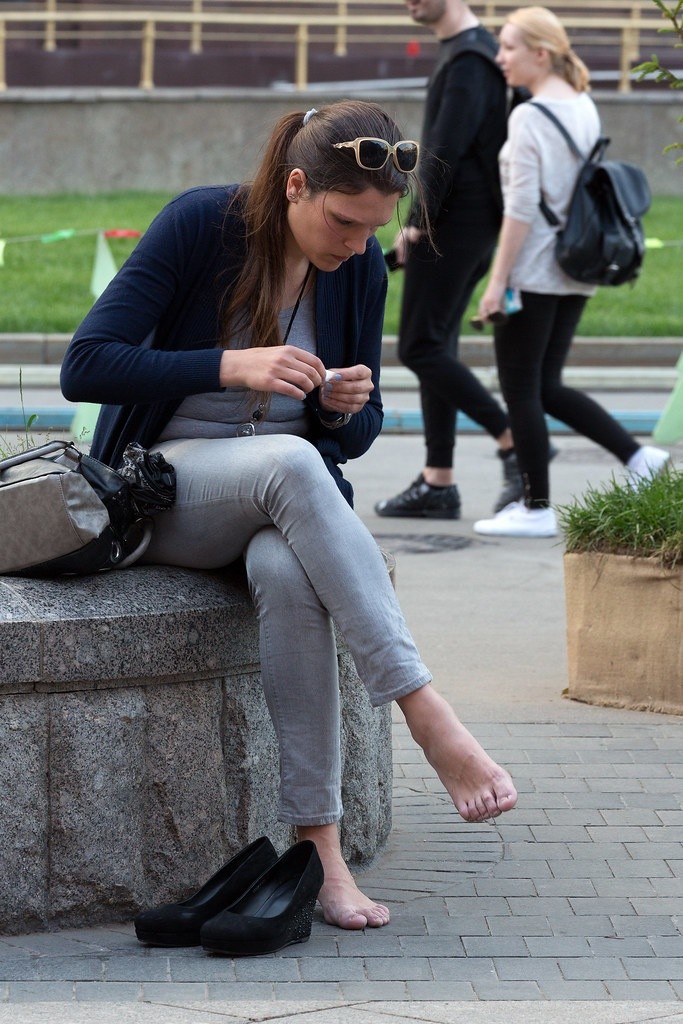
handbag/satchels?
[0,439,153,576]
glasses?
[331,137,421,173]
[469,310,508,331]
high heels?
[134,836,279,947]
[200,839,325,956]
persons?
[392,0,525,520]
[59,100,519,930]
[472,6,671,538]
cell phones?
[385,249,403,274]
[503,285,522,315]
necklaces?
[235,260,313,437]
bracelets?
[317,412,352,430]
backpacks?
[523,101,650,286]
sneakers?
[493,444,560,514]
[473,497,559,537]
[625,446,670,495]
[375,473,462,519]
